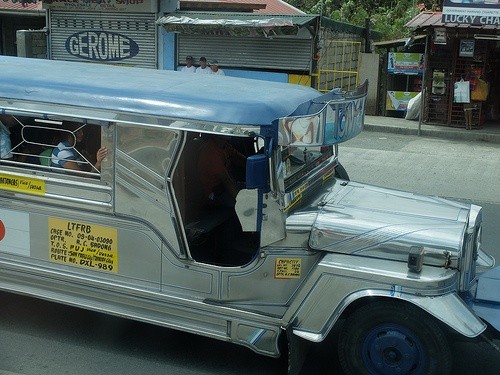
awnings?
[155,11,319,38]
[402,10,500,31]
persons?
[208,62,224,76]
[181,55,196,73]
[196,57,212,74]
[0,114,108,180]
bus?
[0,52,500,375]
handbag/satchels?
[453,79,470,103]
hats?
[208,59,220,66]
[185,56,193,60]
[199,57,206,61]
